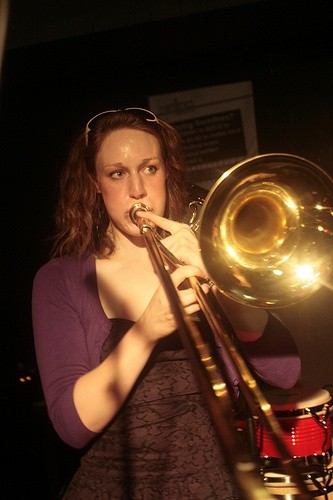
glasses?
[85,108,160,150]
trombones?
[130,152,333,500]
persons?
[31,103,305,500]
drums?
[237,385,333,459]
